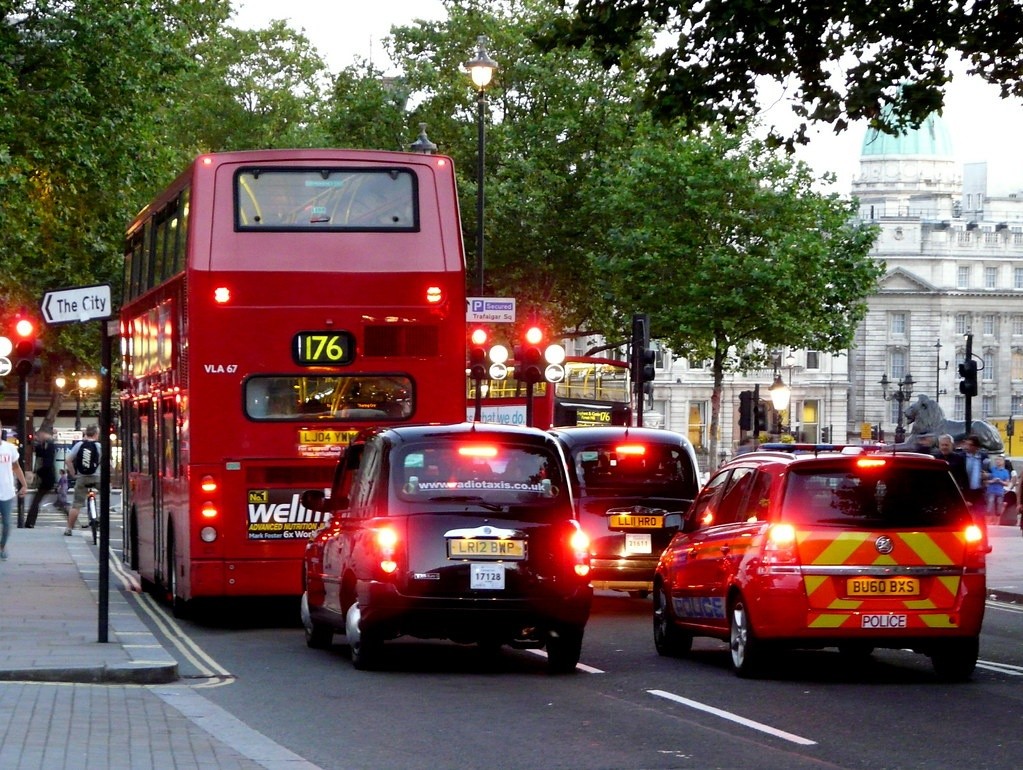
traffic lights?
[632,313,655,383]
[1007,421,1014,435]
[544,342,565,385]
[959,360,978,397]
[791,426,807,443]
[738,390,769,432]
[513,322,543,385]
[466,319,488,379]
[12,305,45,378]
[871,426,884,442]
[488,344,508,381]
[821,427,829,443]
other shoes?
[0,551,9,561]
[64,529,72,536]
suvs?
[301,421,593,671]
[653,444,986,683]
[549,425,701,596]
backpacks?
[76,440,103,475]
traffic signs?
[41,281,111,324]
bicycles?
[82,483,101,545]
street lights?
[463,35,498,297]
[934,339,949,403]
[769,364,793,444]
[57,362,99,431]
[879,373,917,443]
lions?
[877,392,1004,457]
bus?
[120,150,469,618]
[466,354,633,435]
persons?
[983,454,1009,524]
[0,420,28,561]
[995,460,1016,525]
[25,428,69,528]
[732,436,753,459]
[916,428,938,454]
[54,469,67,513]
[958,435,993,553]
[64,424,101,535]
[718,459,727,468]
[930,434,969,497]
